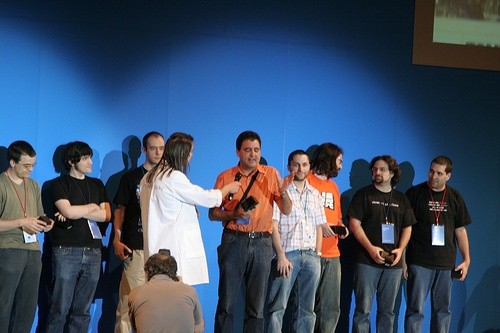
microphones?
[228,172,242,201]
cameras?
[159,249,170,256]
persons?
[112,131,242,333]
[208,130,349,333]
[400,156,473,333]
[345,155,417,333]
[44,141,112,333]
[0,140,55,333]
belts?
[224,229,270,238]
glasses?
[372,168,389,171]
